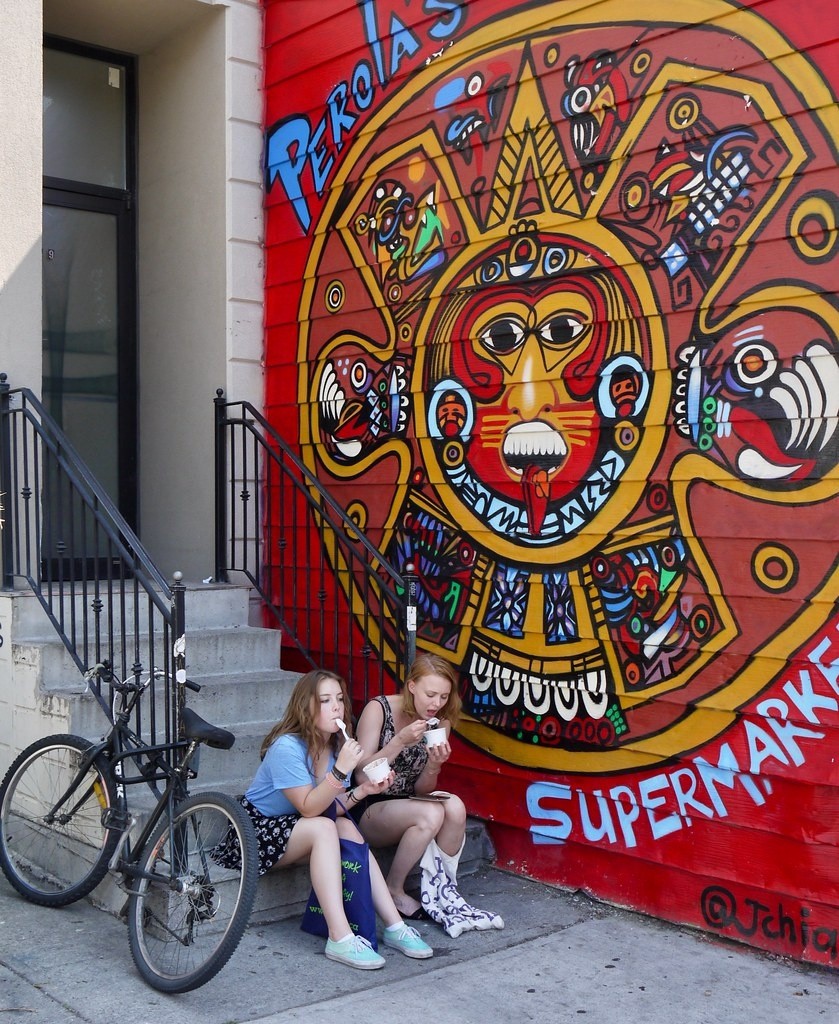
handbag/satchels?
[419,839,505,938]
[300,838,379,952]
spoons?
[336,718,350,742]
[425,718,438,725]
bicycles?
[0,659,262,992]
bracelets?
[345,785,362,803]
[424,764,441,776]
[331,765,348,782]
[330,770,344,783]
[325,772,344,789]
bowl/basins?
[425,727,449,748]
[363,756,391,784]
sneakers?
[325,933,385,970]
[383,925,433,958]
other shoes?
[397,906,432,921]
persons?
[209,669,434,971]
[342,651,467,921]
